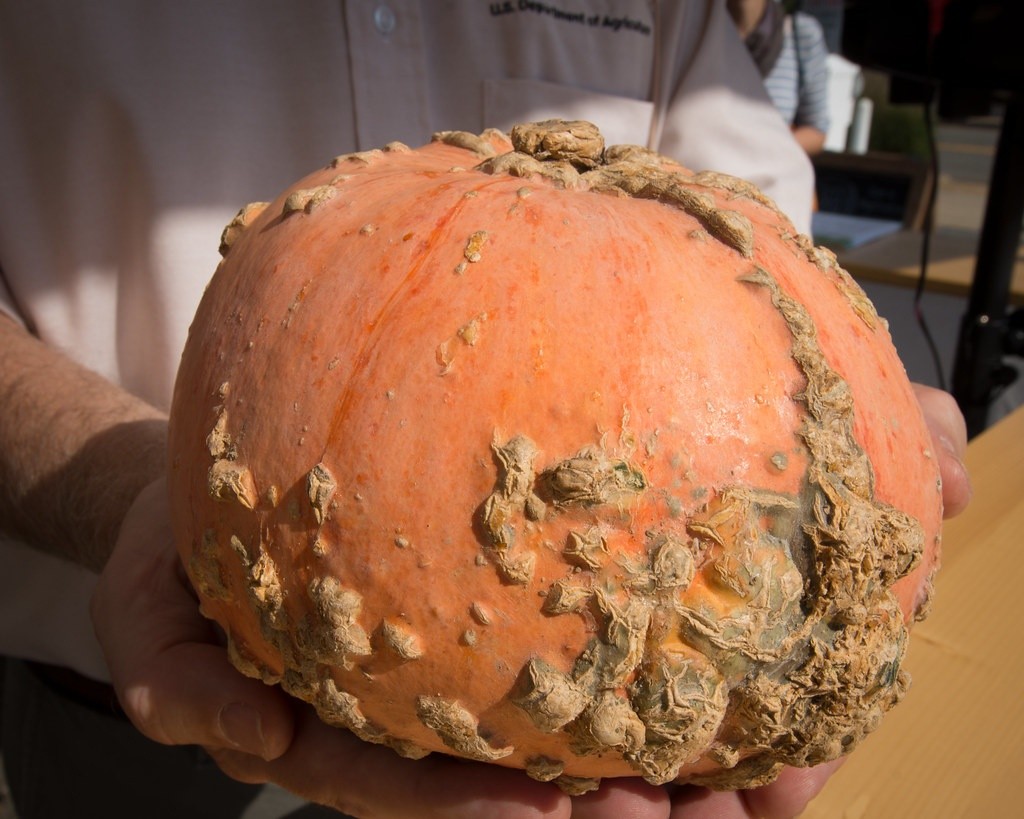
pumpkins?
[165,117,945,796]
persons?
[722,0,840,210]
[1,0,974,817]
